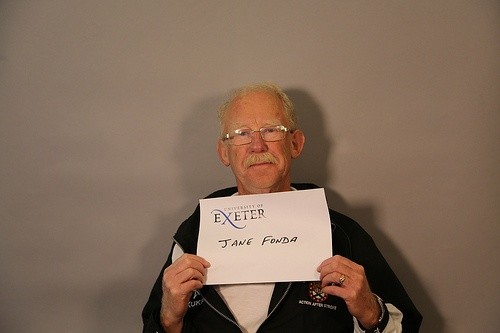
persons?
[141,83,424,333]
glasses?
[222,125,293,146]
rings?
[338,273,346,286]
